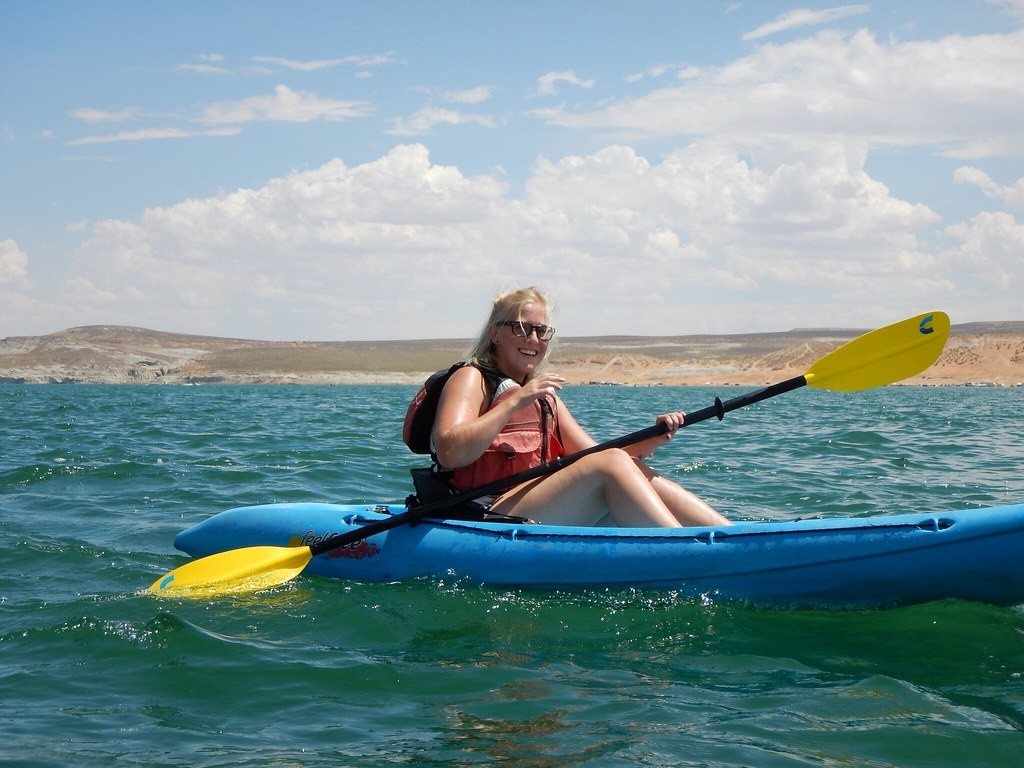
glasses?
[495,320,556,342]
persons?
[432,288,734,529]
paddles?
[149,310,949,600]
